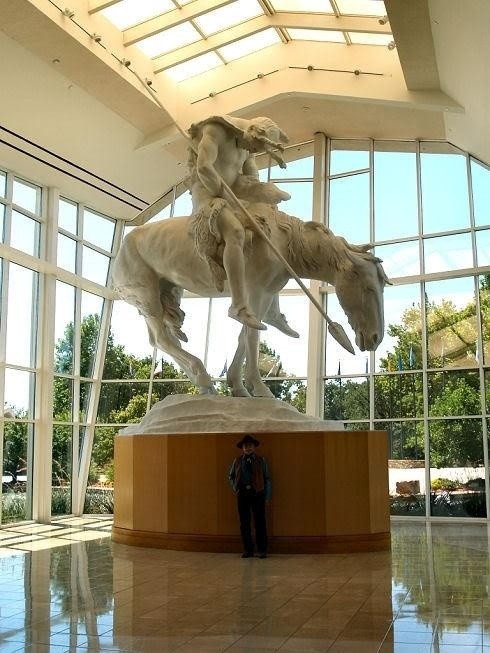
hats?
[237,434,259,449]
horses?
[106,200,395,399]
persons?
[185,112,301,337]
[231,555,274,652]
[229,433,273,558]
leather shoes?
[241,553,267,559]
